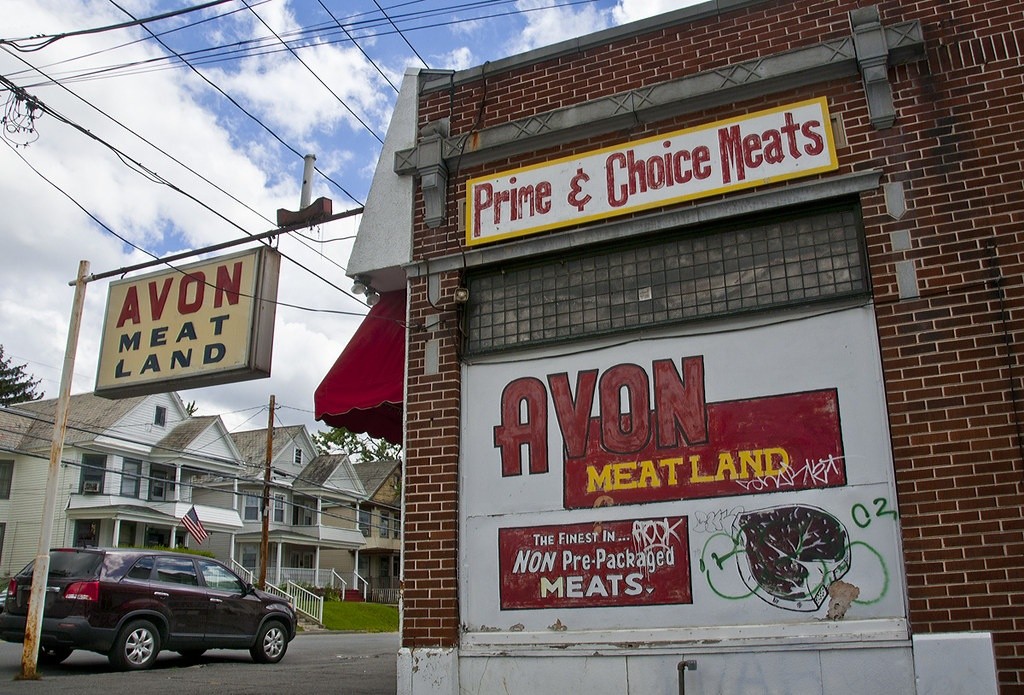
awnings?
[313,289,408,446]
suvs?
[2,545,297,672]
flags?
[182,507,209,546]
